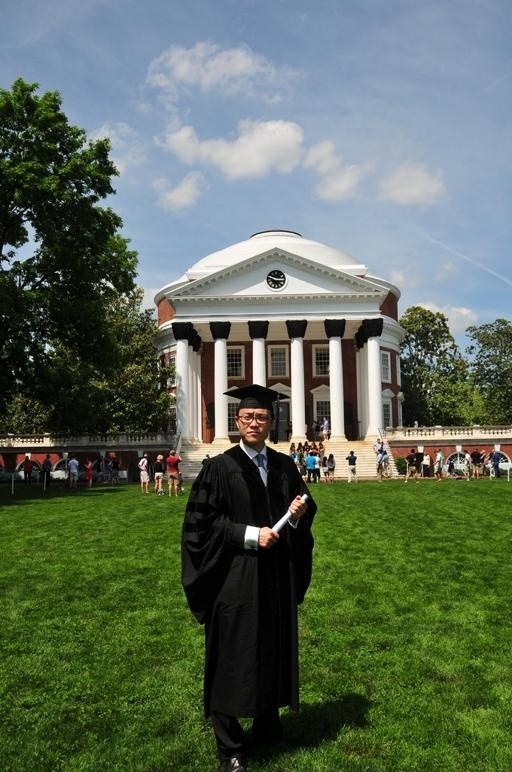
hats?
[222,384,290,444]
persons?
[67,455,122,491]
[152,454,165,495]
[288,441,336,485]
[20,456,33,486]
[373,439,502,484]
[41,454,52,488]
[137,453,151,494]
[346,451,358,483]
[201,453,211,466]
[166,449,183,498]
[178,383,322,772]
[306,416,331,441]
[284,420,293,442]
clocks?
[266,269,288,291]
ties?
[257,454,268,488]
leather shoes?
[226,755,247,772]
